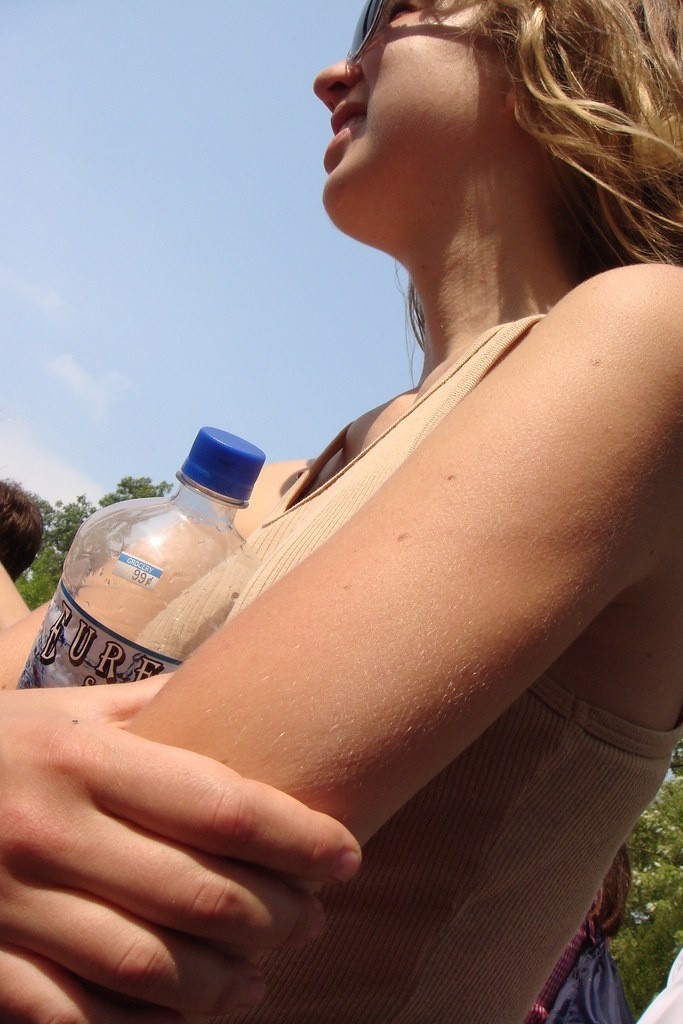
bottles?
[15,425,267,690]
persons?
[0,0,683,1023]
[521,843,635,1024]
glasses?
[346,0,386,67]
